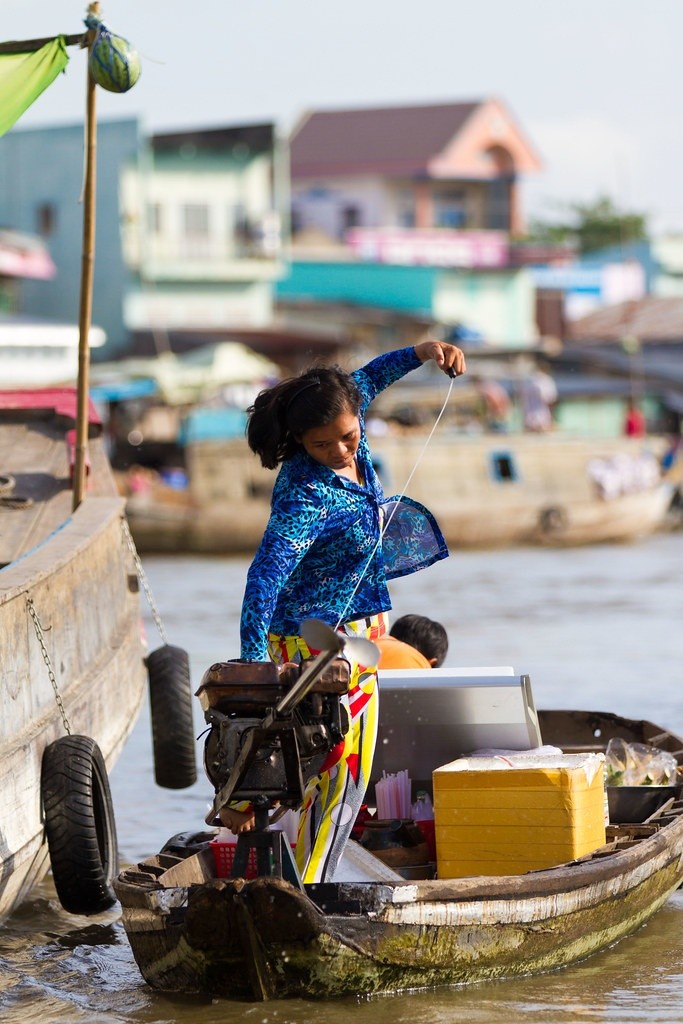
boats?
[117,429,683,551]
[1,3,195,921]
[115,367,683,1001]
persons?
[365,612,450,669]
[214,337,469,886]
